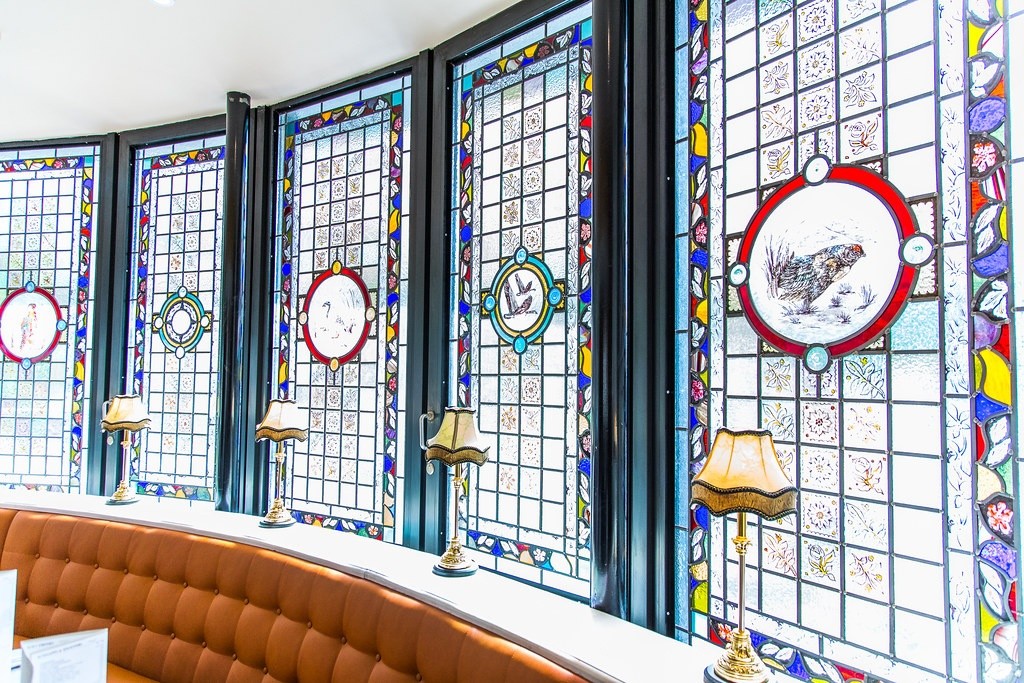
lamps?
[101,393,153,504]
[690,428,800,683]
[425,408,492,578]
[255,401,309,528]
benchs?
[0,509,585,683]
